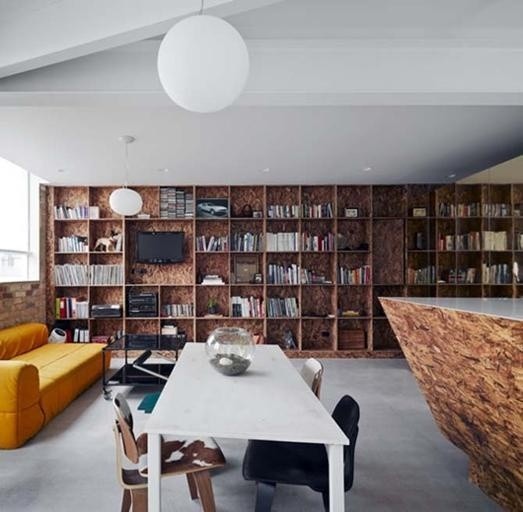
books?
[127,292,193,350]
[267,262,300,318]
[55,263,123,285]
[55,297,123,343]
[196,230,299,251]
[231,296,264,317]
[301,266,369,284]
[201,274,226,285]
[268,205,300,219]
[159,188,194,218]
[57,234,88,251]
[407,202,523,285]
[55,205,89,219]
[302,204,335,251]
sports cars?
[197,202,227,215]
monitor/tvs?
[135,231,185,264]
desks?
[138,340,352,512]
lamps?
[107,134,144,216]
[154,1,251,114]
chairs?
[109,388,227,510]
[300,355,324,399]
[241,393,359,511]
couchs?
[0,324,110,448]
